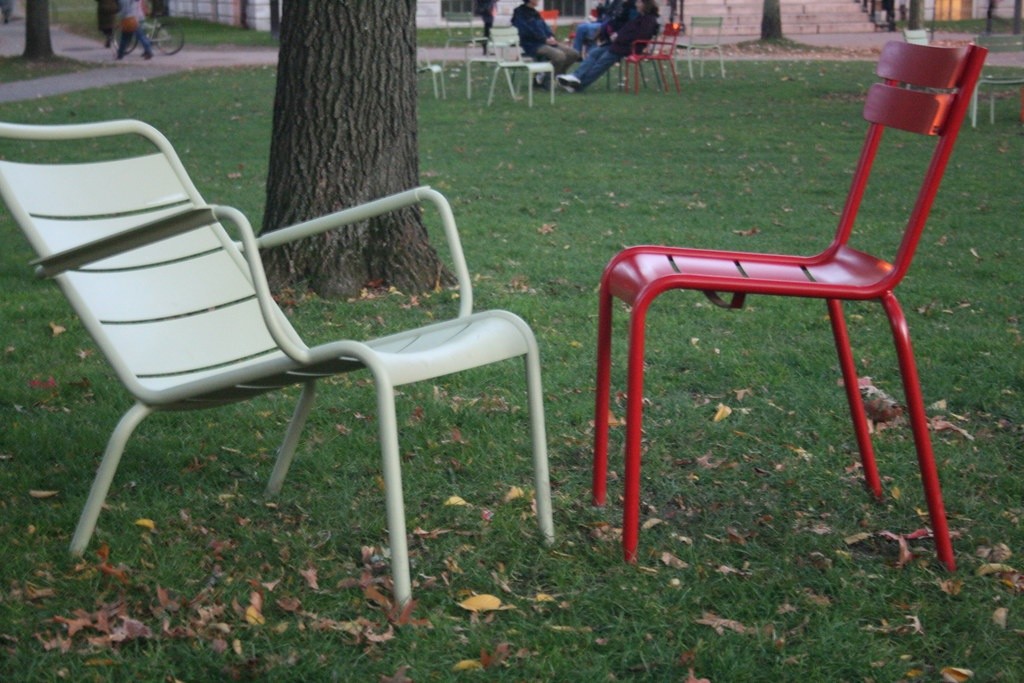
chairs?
[592,41,989,574]
[416,10,725,107]
[903,25,1023,128]
[0,119,555,611]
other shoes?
[535,72,546,86]
[556,74,581,94]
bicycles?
[110,8,185,55]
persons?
[511,1,580,90]
[95,0,120,48]
[556,0,660,93]
[114,1,154,60]
[473,0,497,56]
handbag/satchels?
[119,16,139,32]
